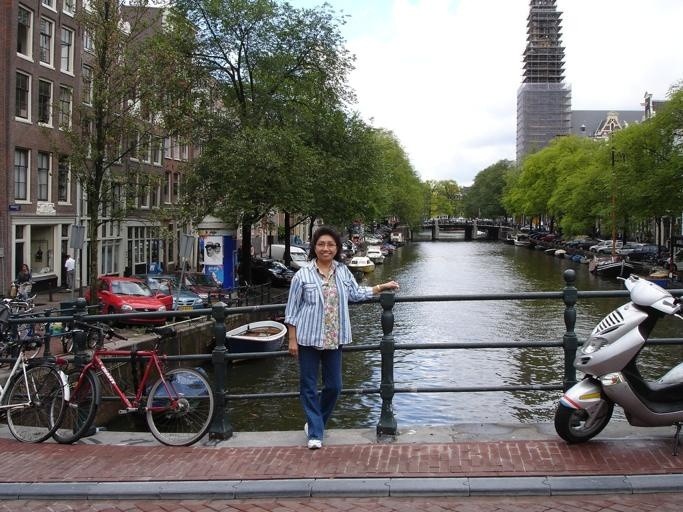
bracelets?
[376,284,382,292]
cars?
[171,269,230,308]
[130,273,202,320]
[237,220,382,288]
[82,274,172,328]
[518,222,682,272]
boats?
[502,230,530,247]
[347,228,408,275]
[136,366,210,418]
[644,270,678,290]
[589,260,633,277]
[205,315,287,359]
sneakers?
[303,421,308,437]
[307,439,321,449]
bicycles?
[0,340,70,444]
[47,319,215,446]
[0,292,37,345]
[60,303,100,354]
[0,300,43,369]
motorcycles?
[554,260,682,457]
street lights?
[580,125,616,256]
[335,117,374,250]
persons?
[237,243,255,263]
[282,228,401,450]
[146,257,165,282]
[64,255,77,291]
[205,241,222,265]
[15,263,33,302]
